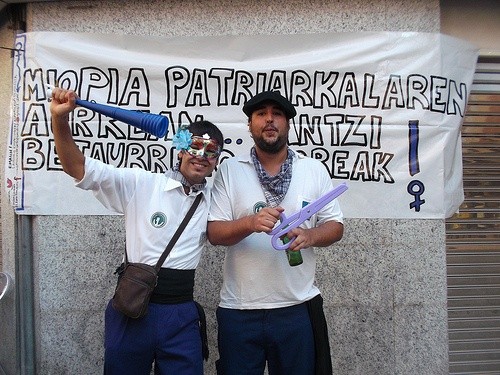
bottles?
[280,217,303,266]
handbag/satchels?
[109,260,159,320]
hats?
[242,90,298,120]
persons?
[49,87,223,375]
[207,91,344,375]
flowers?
[172,128,194,150]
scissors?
[266,185,350,251]
